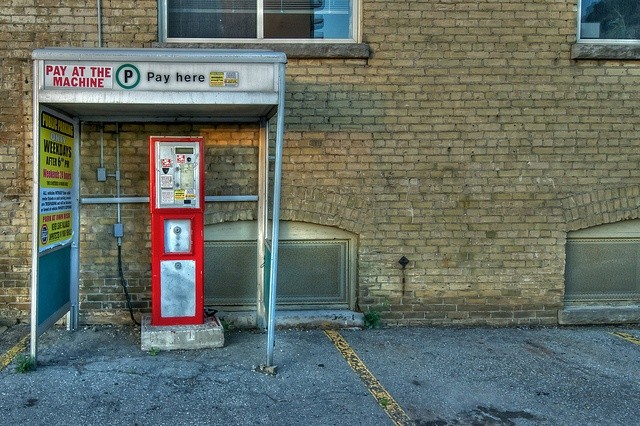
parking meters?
[149,135,204,325]
